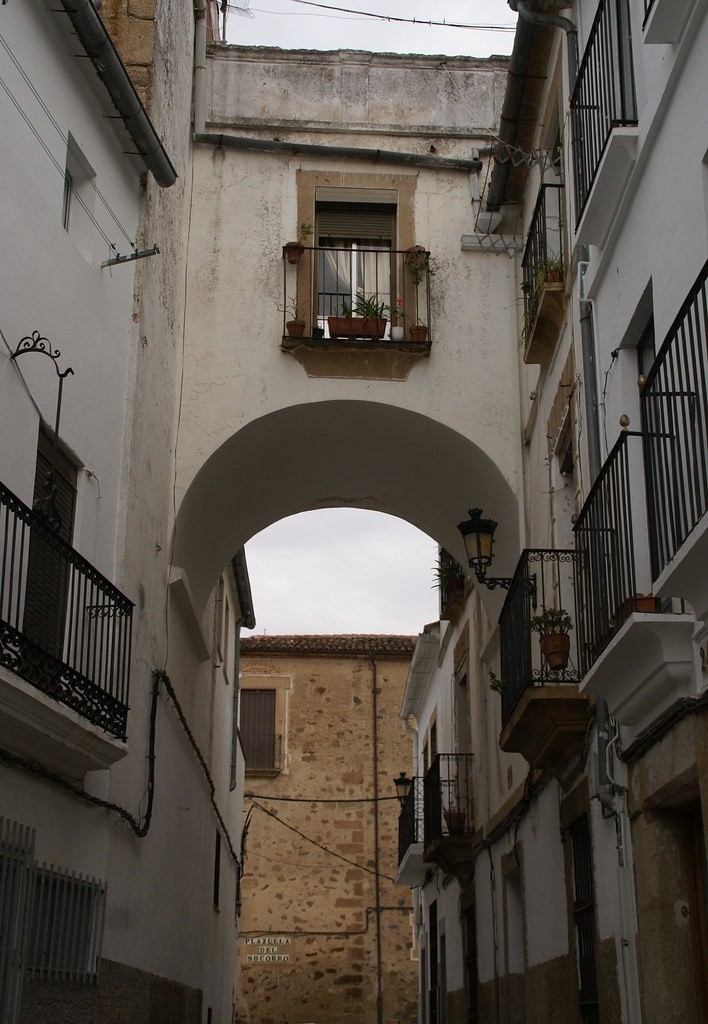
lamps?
[457,507,538,614]
[393,771,412,808]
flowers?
[391,296,411,326]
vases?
[390,327,403,341]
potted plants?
[530,246,568,283]
[310,321,324,339]
[613,592,662,630]
[442,793,473,833]
[526,608,574,671]
[404,245,436,286]
[286,223,317,264]
[328,290,401,338]
[274,297,309,336]
[410,318,428,341]
[584,626,617,656]
